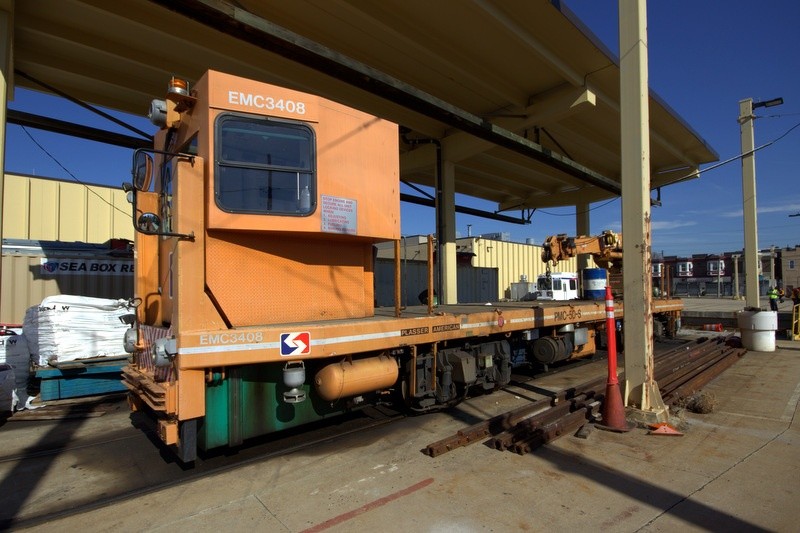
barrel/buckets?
[582,268,607,301]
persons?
[778,288,785,303]
[767,285,779,311]
[791,287,800,312]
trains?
[122,68,687,463]
